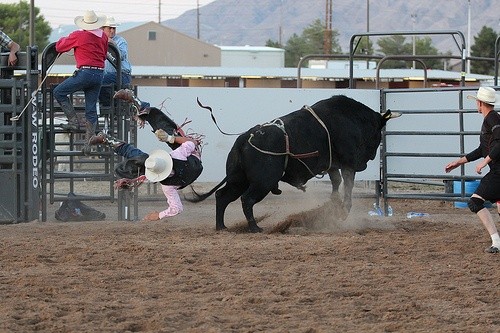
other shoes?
[484,245,499,254]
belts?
[80,66,103,70]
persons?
[87,89,208,223]
[444,87,500,254]
[97,16,151,120]
[54,9,108,155]
[0,28,21,68]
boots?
[59,101,81,133]
[89,131,128,153]
[83,119,98,156]
[113,89,151,115]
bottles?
[121,205,128,220]
[367,202,393,216]
[404,212,429,218]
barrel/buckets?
[442,179,481,209]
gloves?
[155,129,175,144]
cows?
[184,95,402,233]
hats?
[74,10,106,30]
[467,86,500,105]
[145,149,173,182]
[102,16,119,27]
